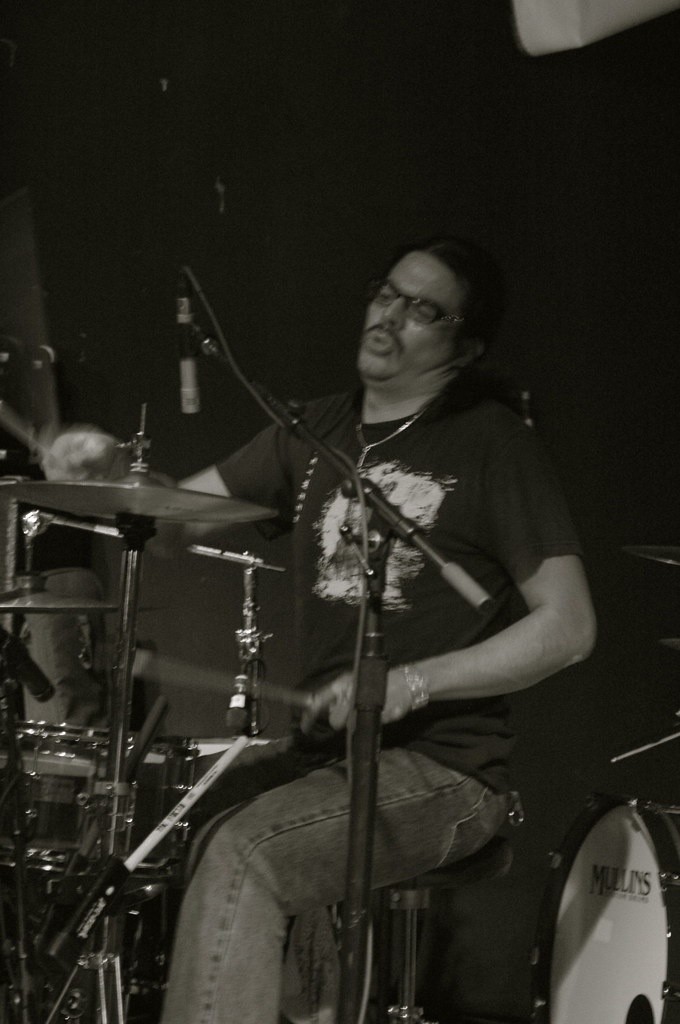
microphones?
[175,273,200,414]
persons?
[1,240,598,1023]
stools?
[386,835,512,1024]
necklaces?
[355,409,428,469]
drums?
[529,790,680,1024]
[0,716,193,878]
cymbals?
[621,544,679,565]
[0,473,278,524]
[0,586,172,614]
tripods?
[42,518,159,1024]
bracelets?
[400,664,430,714]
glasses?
[366,278,468,326]
